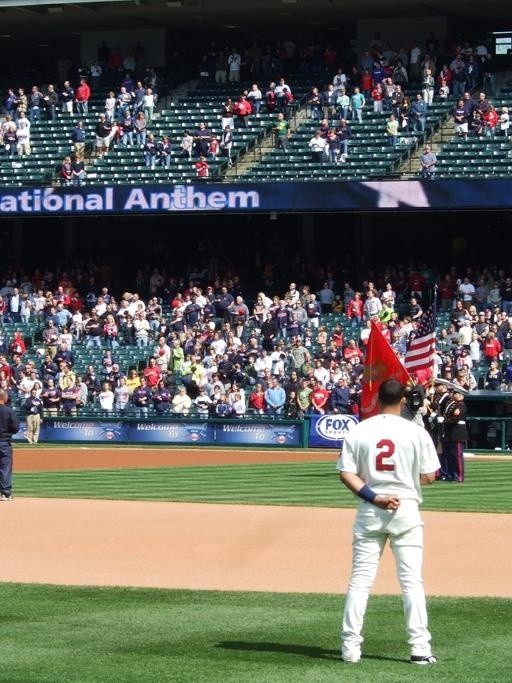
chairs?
[1,60,512,189]
[1,290,512,420]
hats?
[448,383,468,395]
[435,378,449,385]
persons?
[0,259,512,501]
[2,30,511,185]
[335,378,442,665]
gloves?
[419,398,444,424]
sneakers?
[410,656,438,665]
[29,442,37,444]
[0,495,13,500]
